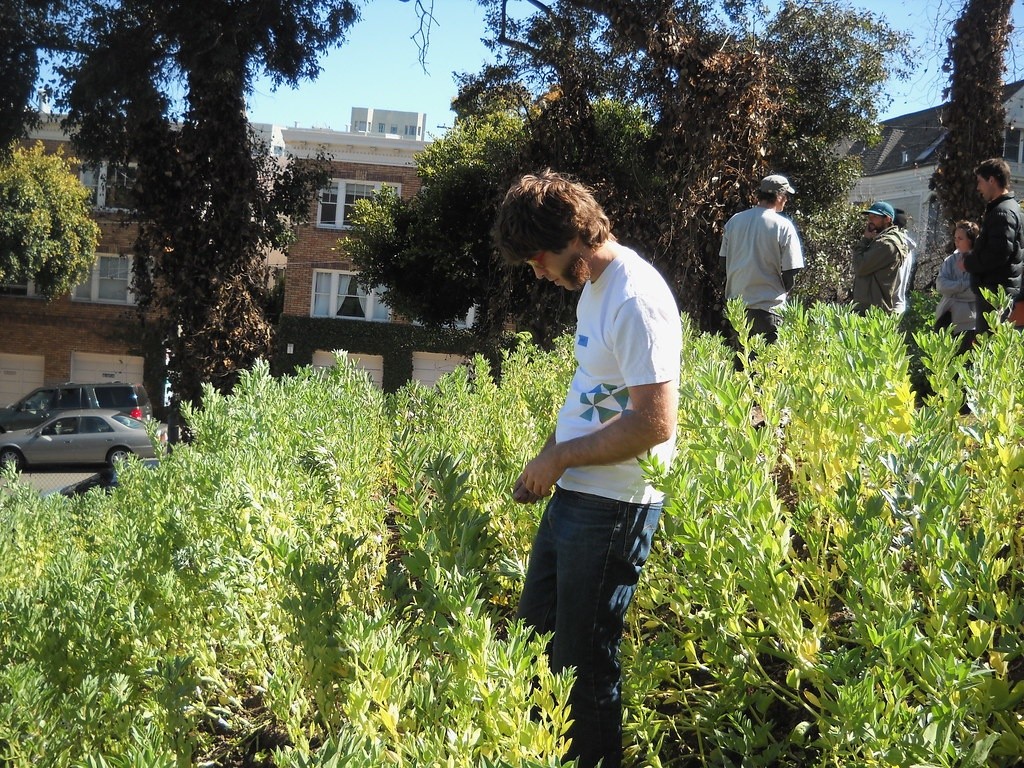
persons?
[494,172,682,768]
[718,175,805,371]
[934,160,1024,415]
[853,201,916,320]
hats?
[860,201,895,221]
[761,175,795,194]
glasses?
[525,250,545,270]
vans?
[0,381,153,436]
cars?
[0,409,169,473]
